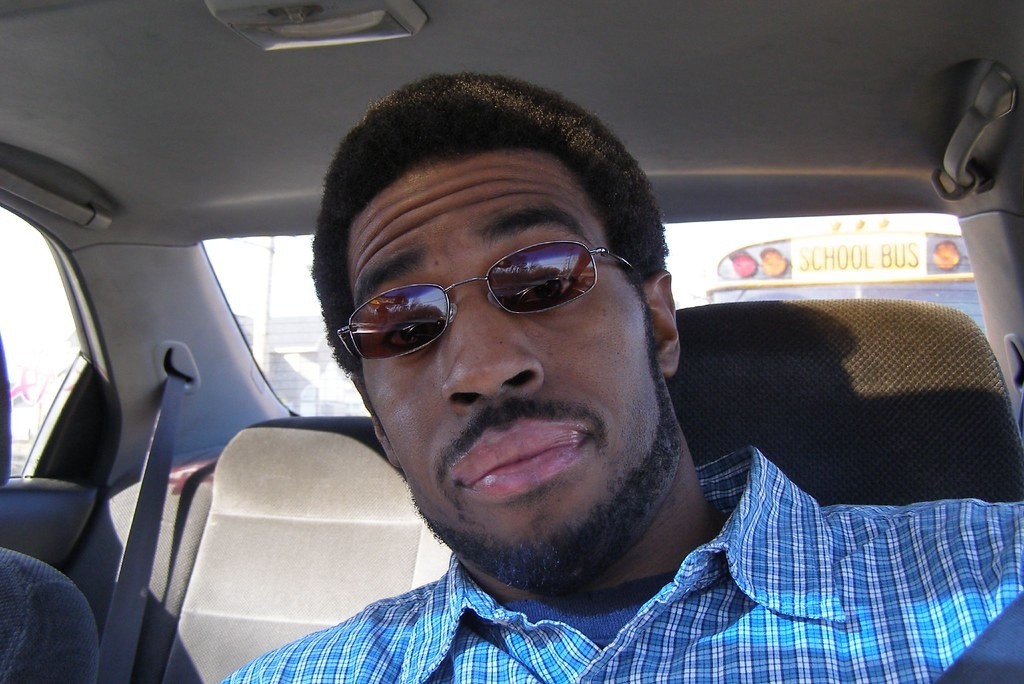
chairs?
[666,298,1024,506]
[163,416,452,684]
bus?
[707,216,988,335]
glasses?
[336,241,637,362]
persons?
[217,68,1024,684]
